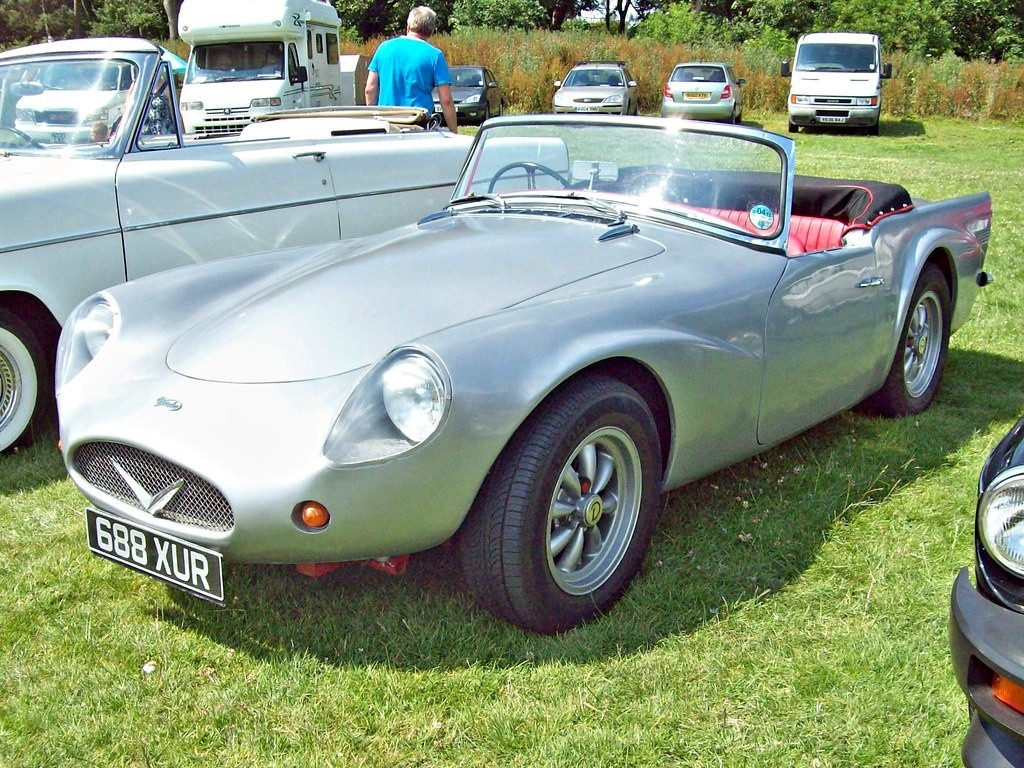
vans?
[781,32,892,136]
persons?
[123,76,184,135]
[90,122,108,142]
[365,6,458,133]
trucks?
[177,0,343,137]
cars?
[948,414,1024,768]
[0,35,572,457]
[430,66,504,123]
[14,63,139,144]
[55,112,991,636]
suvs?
[661,62,746,124]
[551,61,640,117]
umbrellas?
[159,45,187,74]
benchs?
[242,117,398,135]
[682,207,849,258]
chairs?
[578,73,591,84]
[608,76,619,85]
[787,232,805,255]
[472,74,481,82]
[713,71,723,80]
[685,72,693,80]
